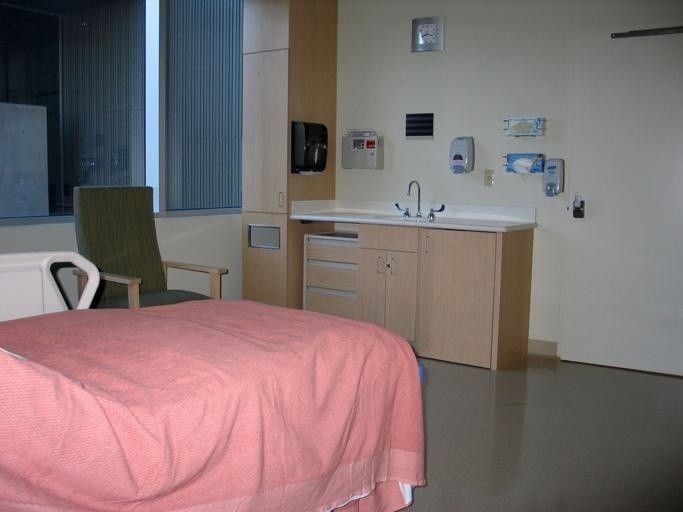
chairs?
[65,183,230,307]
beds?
[0,293,428,507]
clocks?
[410,16,445,55]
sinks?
[386,217,430,221]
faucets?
[406,178,421,217]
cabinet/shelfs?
[415,229,534,369]
[353,224,417,355]
[302,235,359,322]
[241,0,338,302]
